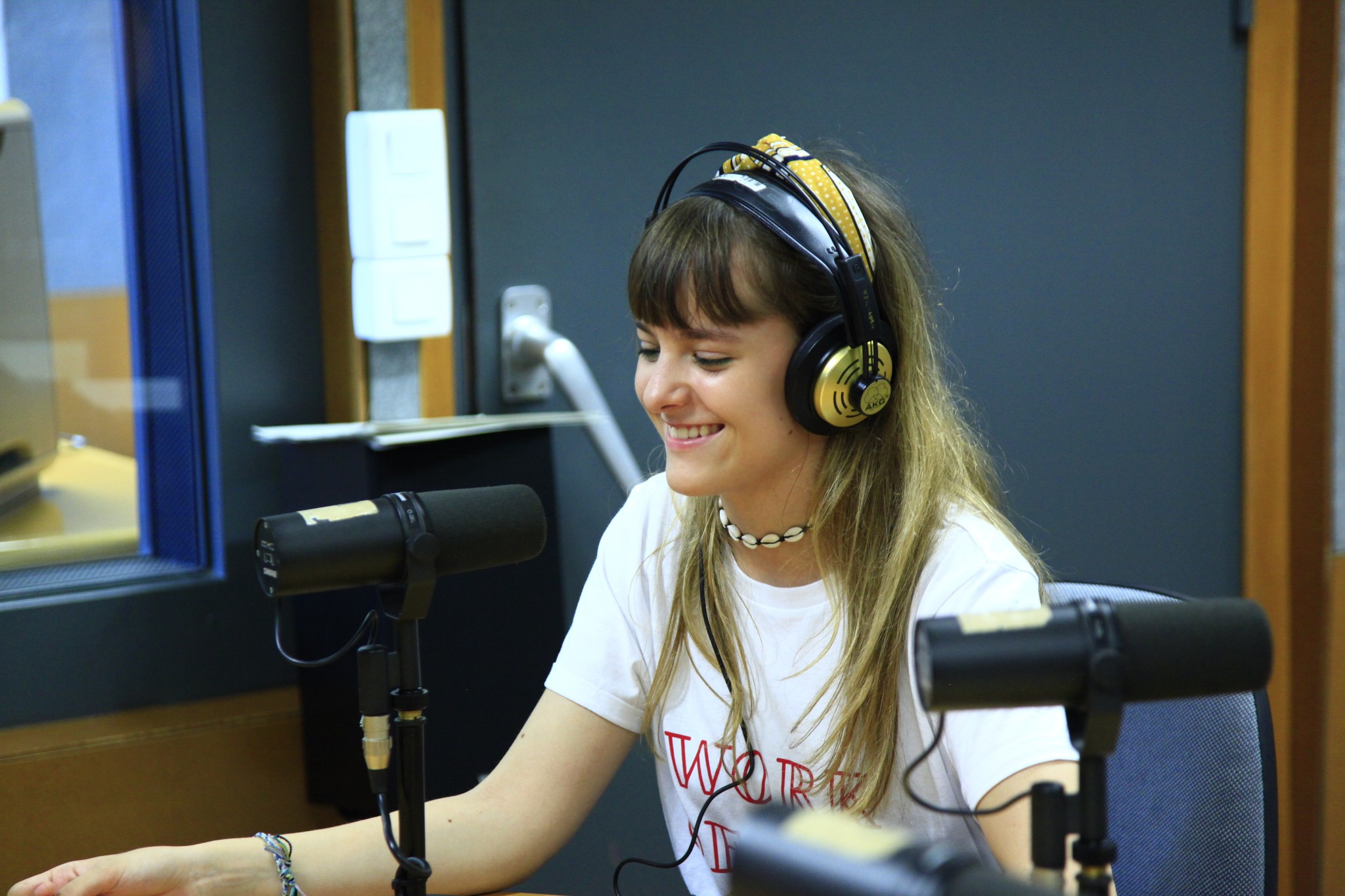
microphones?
[252,487,546,601]
[914,594,1271,709]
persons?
[6,154,1118,896]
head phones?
[644,131,897,437]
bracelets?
[253,832,305,896]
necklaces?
[718,498,830,549]
